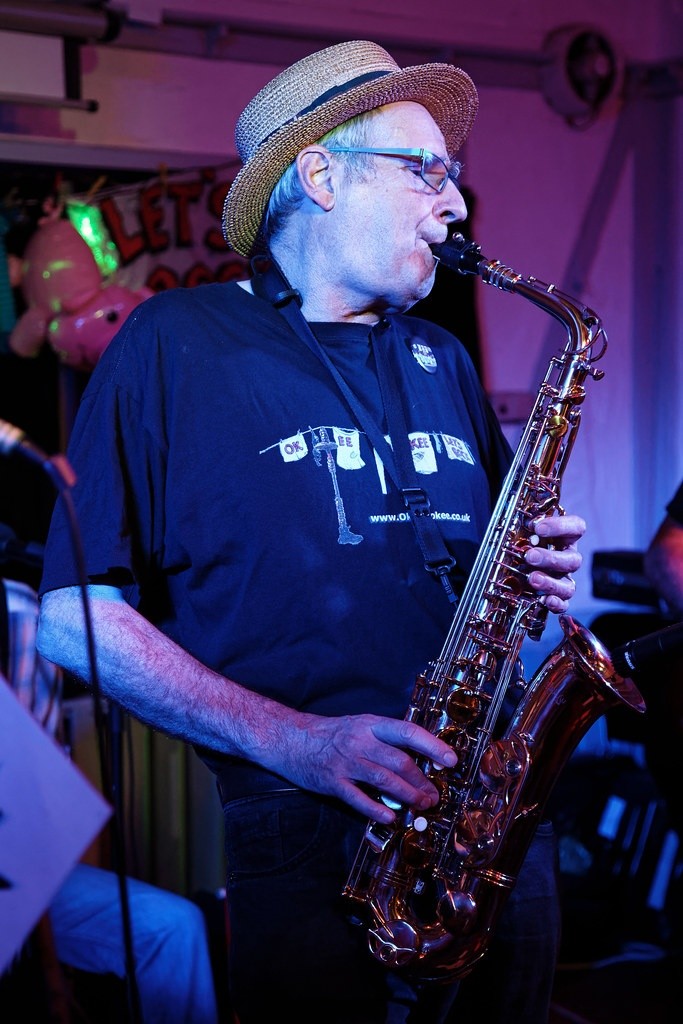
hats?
[220,39,481,262]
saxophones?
[336,232,648,987]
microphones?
[614,622,683,679]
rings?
[569,576,579,597]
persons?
[36,40,586,1024]
[2,862,217,1024]
[644,480,683,611]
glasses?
[325,145,461,195]
